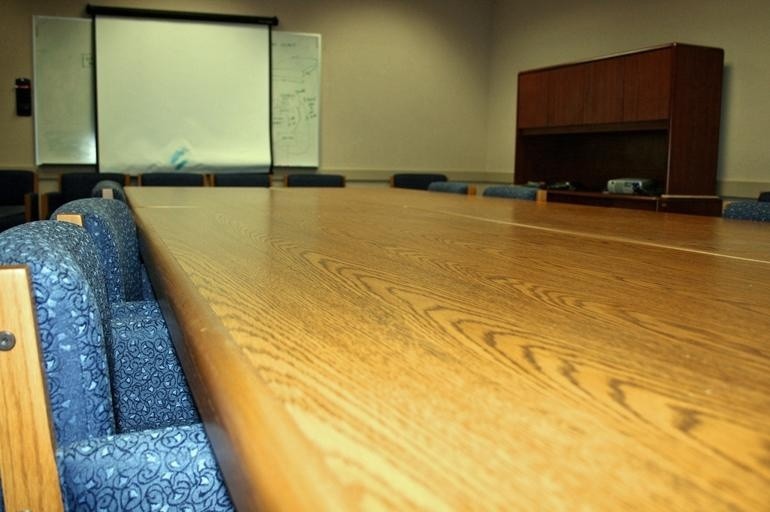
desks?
[117,183,770,512]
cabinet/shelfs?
[514,39,726,220]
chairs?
[389,173,447,193]
[206,172,273,189]
[1,169,40,219]
[48,195,158,303]
[138,172,205,187]
[1,218,239,510]
[724,199,768,221]
[427,182,477,197]
[482,184,547,204]
[58,171,129,211]
[282,172,346,188]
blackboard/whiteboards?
[34,16,322,165]
